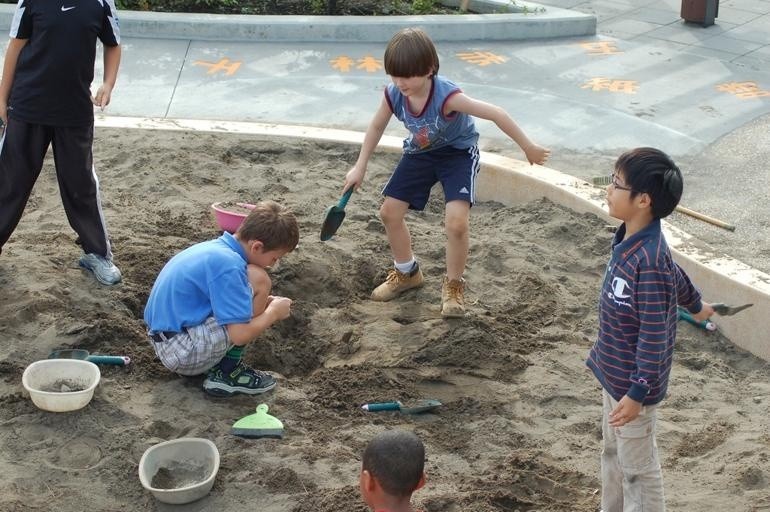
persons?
[356,426,429,511]
[338,27,551,321]
[582,144,715,510]
[141,200,300,400]
[0,0,126,288]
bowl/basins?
[212,201,257,232]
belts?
[152,332,176,341]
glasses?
[612,173,633,191]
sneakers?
[440,277,466,317]
[79,253,121,285]
[371,262,425,302]
[202,359,276,396]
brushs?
[229,401,286,437]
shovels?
[711,302,756,316]
[361,397,444,414]
[319,186,355,242]
[45,348,133,367]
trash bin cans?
[682,1,718,25]
